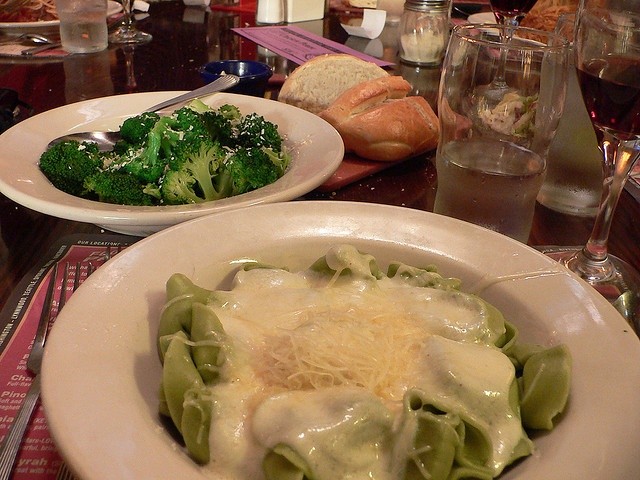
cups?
[535,11,640,218]
[62,47,115,105]
[197,58,274,97]
[431,24,571,244]
[55,0,110,54]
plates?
[0,89,346,238]
[0,0,124,37]
[38,199,640,480]
[467,10,573,52]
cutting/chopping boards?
[315,114,473,194]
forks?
[0,260,94,480]
[104,244,131,263]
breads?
[276,51,442,163]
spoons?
[0,32,53,44]
[612,289,640,340]
[47,73,241,155]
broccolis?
[38,97,293,207]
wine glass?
[530,0,640,305]
[107,0,154,44]
[109,36,153,95]
[471,0,539,100]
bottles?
[396,0,451,69]
[258,43,277,73]
[254,0,285,27]
